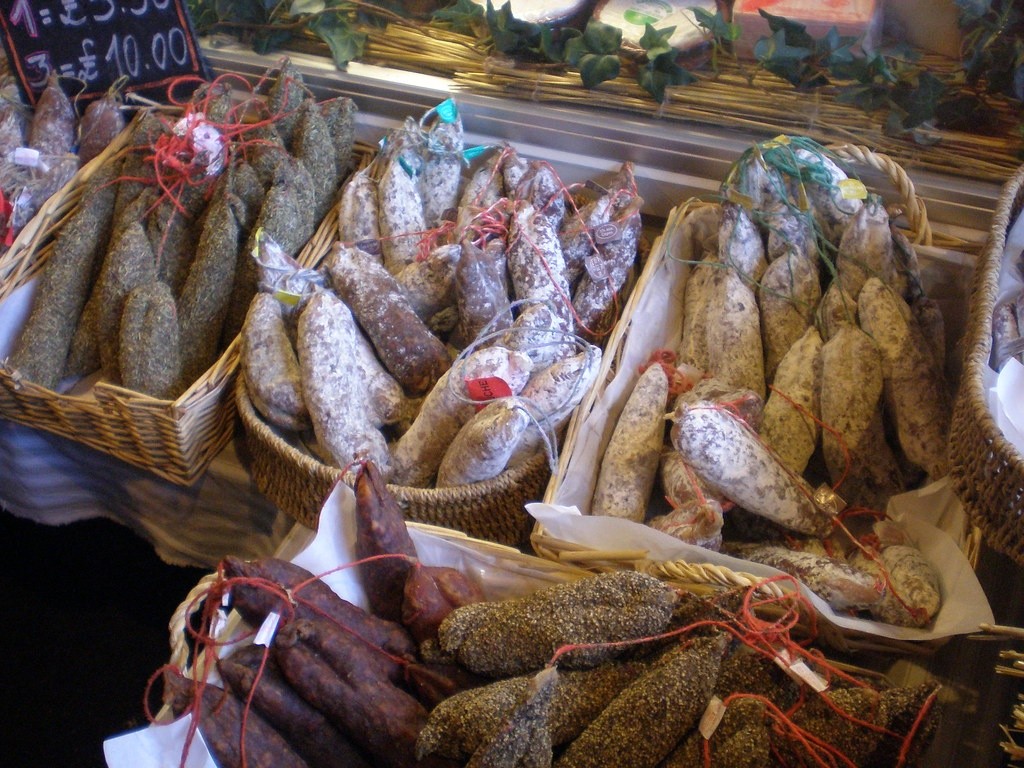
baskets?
[151,521,1024,768]
[528,195,983,658]
[0,104,376,488]
[235,190,650,546]
[945,164,1024,570]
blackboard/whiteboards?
[0,0,214,151]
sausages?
[0,53,977,768]
